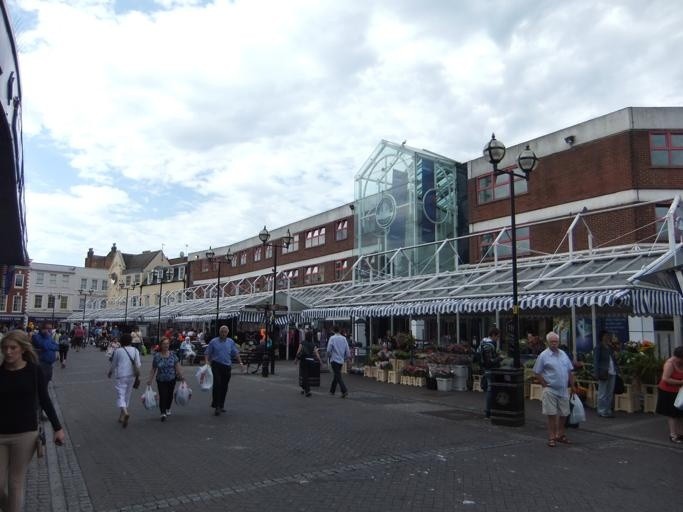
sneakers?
[670,435,683,443]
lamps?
[565,135,576,143]
[350,204,354,209]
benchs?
[178,348,210,365]
[232,349,275,375]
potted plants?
[363,334,408,385]
[523,350,660,413]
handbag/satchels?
[614,374,624,394]
[297,342,303,359]
[133,363,140,375]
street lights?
[118,279,135,329]
[78,288,93,325]
[150,267,173,342]
[483,129,539,366]
[258,225,293,345]
[205,246,234,337]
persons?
[26,320,87,422]
[293,332,324,397]
[655,346,683,444]
[532,332,576,445]
[0,330,65,512]
[204,325,243,416]
[323,326,352,399]
[89,322,204,366]
[105,334,140,428]
[590,330,617,418]
[479,327,505,422]
[146,337,182,421]
[526,329,545,358]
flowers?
[402,365,451,378]
[427,351,462,363]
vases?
[427,364,469,390]
[400,375,452,391]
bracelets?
[239,362,244,365]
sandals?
[559,435,573,443]
[549,438,556,447]
